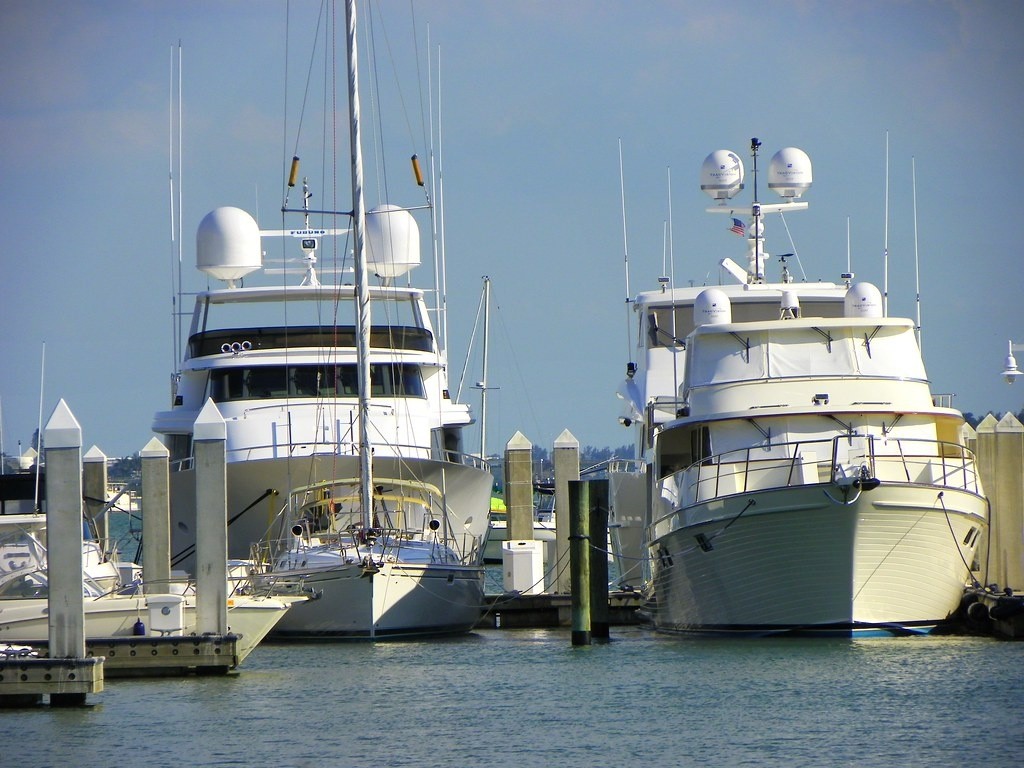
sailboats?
[236,0,498,644]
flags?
[729,217,745,237]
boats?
[608,136,994,637]
[0,341,290,667]
[148,0,499,581]
